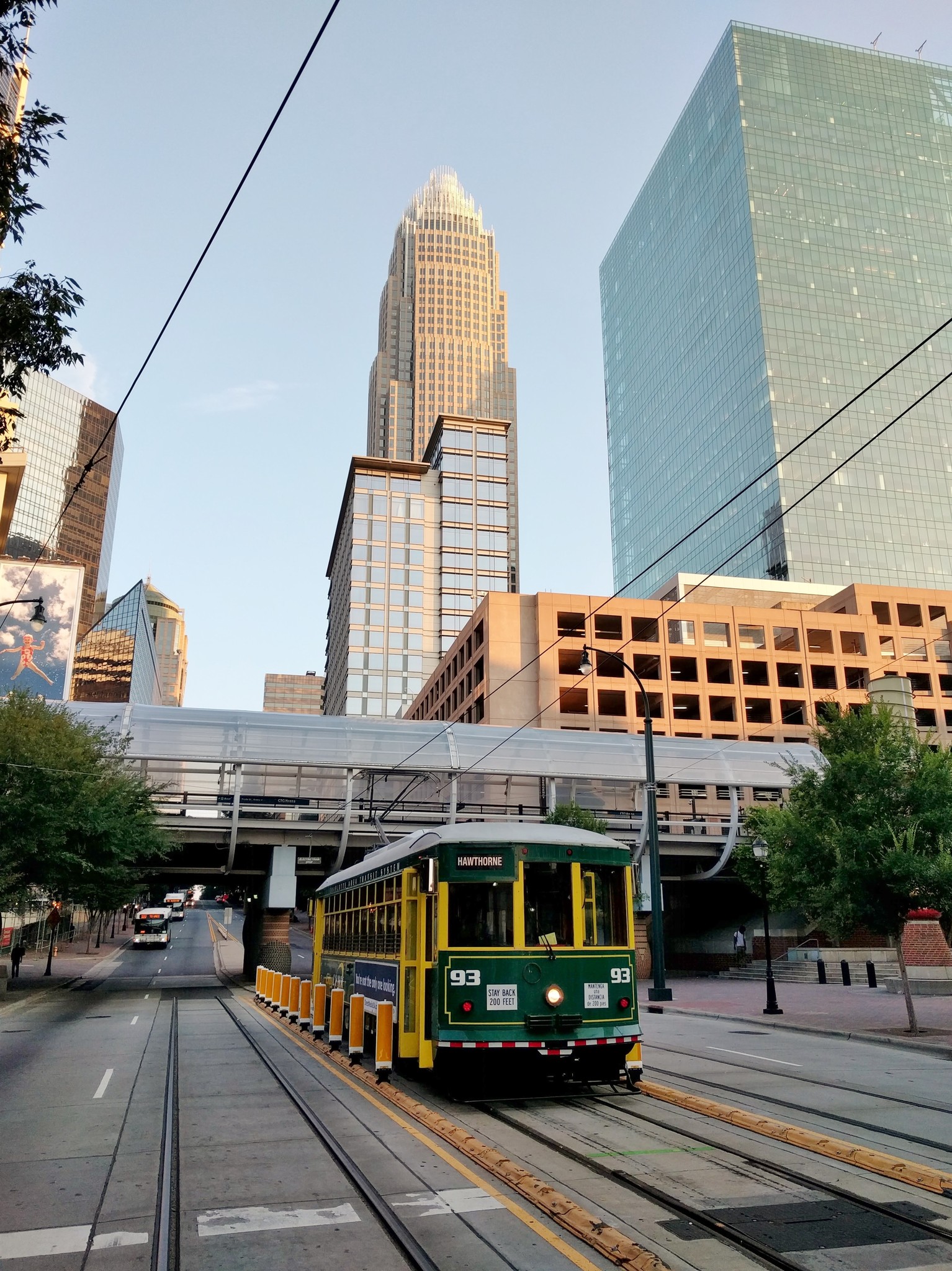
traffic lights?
[51,900,60,910]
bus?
[132,907,172,949]
[178,890,195,906]
[163,893,186,921]
[310,822,646,1103]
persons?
[733,925,746,968]
[0,634,54,686]
[11,943,22,979]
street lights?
[751,835,784,1015]
[580,643,672,1001]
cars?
[217,896,225,903]
[215,895,220,900]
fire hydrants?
[53,945,59,957]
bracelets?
[5,649,6,652]
